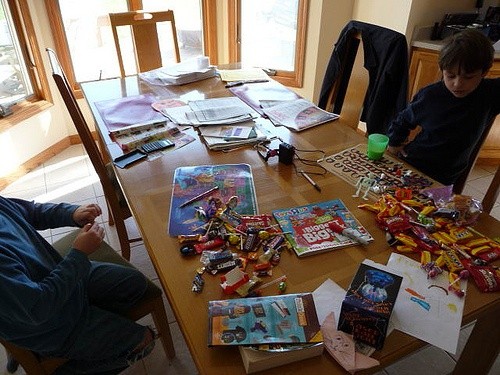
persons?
[387,31,500,195]
[1,192,156,375]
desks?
[80,63,500,375]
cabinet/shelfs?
[406,25,500,163]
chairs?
[109,10,181,77]
[0,228,176,375]
[452,112,500,213]
[324,20,405,137]
[45,48,144,261]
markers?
[329,222,370,246]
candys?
[178,195,288,299]
[358,183,500,297]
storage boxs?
[441,13,491,41]
[337,263,403,350]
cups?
[367,134,389,160]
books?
[156,56,216,84]
[207,292,324,374]
[273,198,375,258]
[219,67,269,81]
[185,97,276,151]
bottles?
[430,23,440,40]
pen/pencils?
[225,82,244,89]
[301,170,321,193]
[178,185,220,208]
